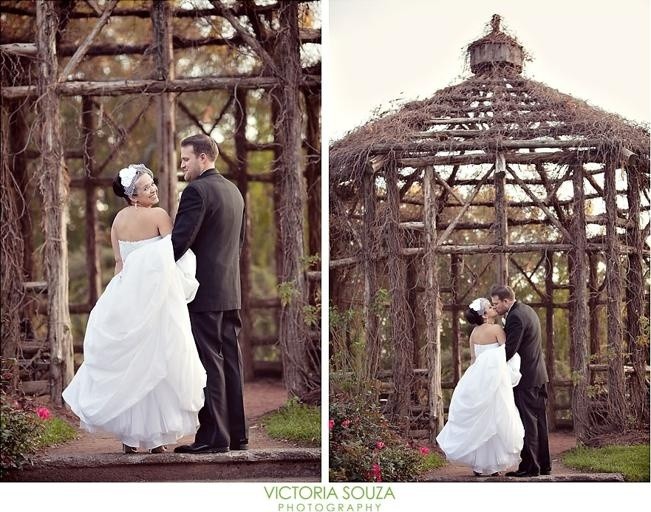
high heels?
[122,443,138,454]
[149,445,167,454]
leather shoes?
[174,442,249,453]
[505,470,551,477]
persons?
[60,162,205,454]
[169,133,251,453]
[434,297,521,479]
[490,285,554,478]
